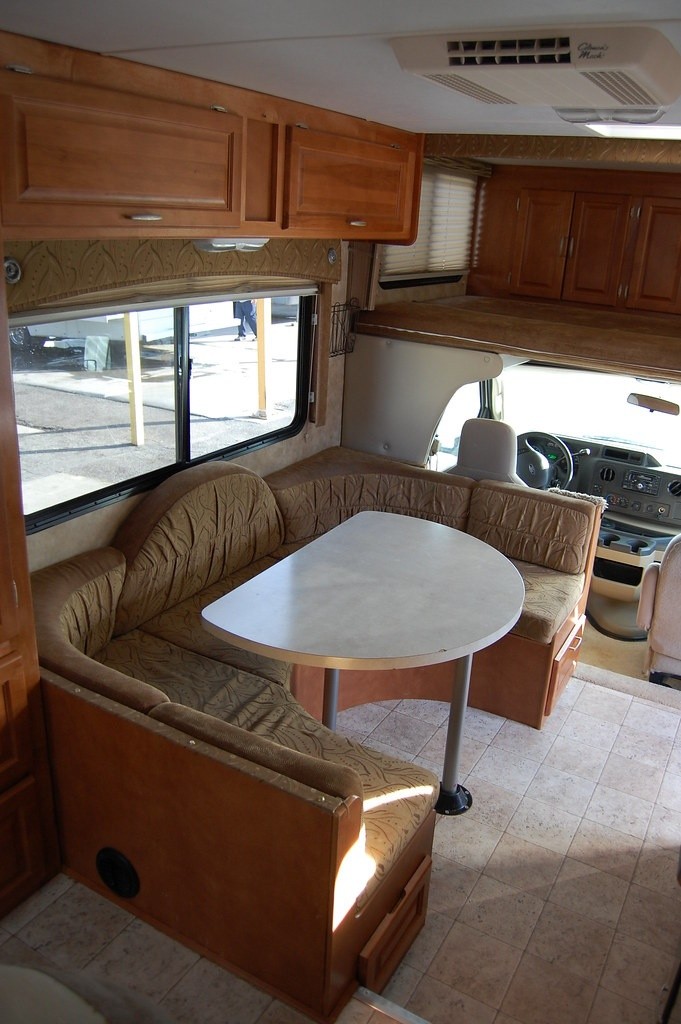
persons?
[233,300,257,341]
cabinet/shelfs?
[0,72,422,245]
[510,185,680,315]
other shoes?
[252,336,257,341]
[234,334,246,341]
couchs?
[30,447,604,1023]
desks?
[201,511,525,816]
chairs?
[636,532,681,693]
[443,417,529,487]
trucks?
[8,298,244,351]
